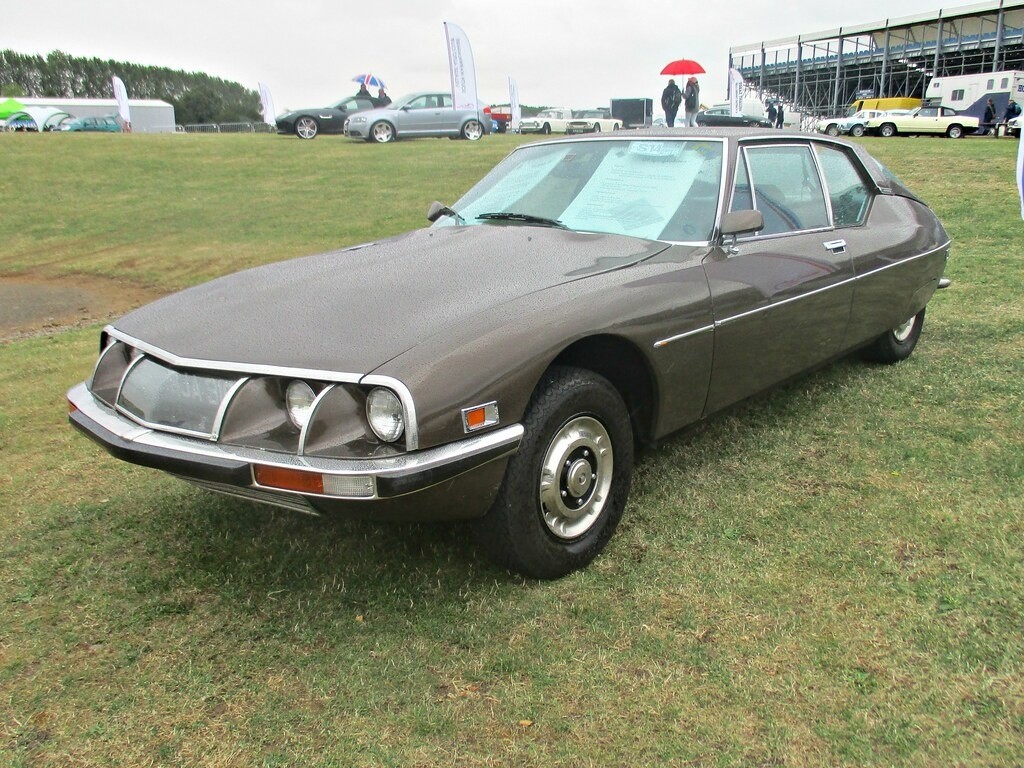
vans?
[841,98,922,119]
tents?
[0,97,30,121]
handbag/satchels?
[685,85,696,109]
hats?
[688,77,697,84]
[1009,99,1014,100]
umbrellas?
[660,58,706,90]
[352,73,388,91]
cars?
[1007,107,1024,138]
[815,106,980,139]
[65,124,951,579]
[62,115,123,132]
[274,96,389,139]
[343,93,492,141]
[695,109,773,128]
[519,108,623,135]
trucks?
[926,70,1024,135]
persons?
[661,79,681,127]
[1003,100,1022,135]
[378,89,392,103]
[766,102,784,128]
[682,78,700,127]
[982,99,996,135]
[356,84,372,96]
